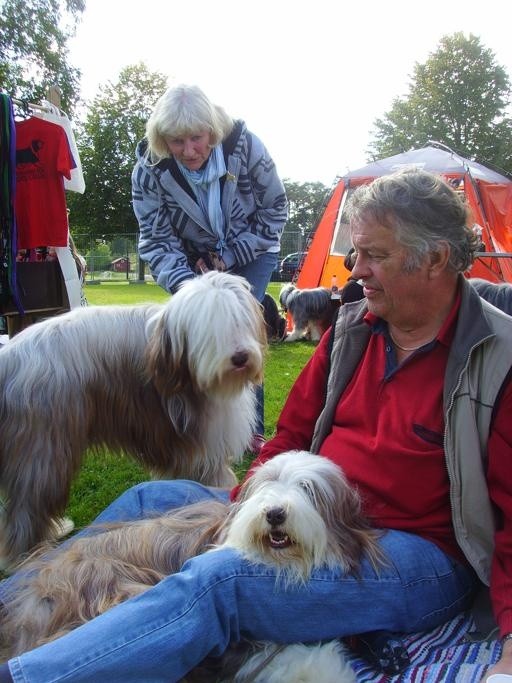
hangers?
[13,99,40,124]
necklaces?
[388,332,437,351]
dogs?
[0,265,270,576]
[1,445,408,682]
[341,278,512,316]
[278,283,341,344]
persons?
[0,166,512,682]
[130,80,289,453]
[341,248,367,305]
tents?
[286,148,512,334]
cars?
[279,251,309,283]
[269,255,289,280]
